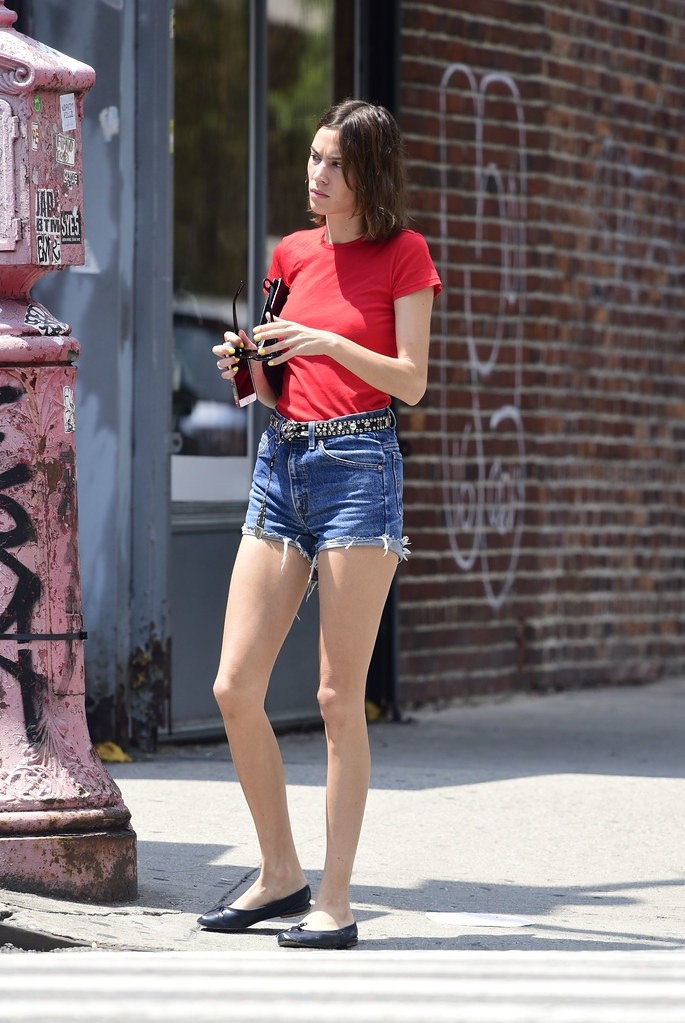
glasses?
[233,281,281,361]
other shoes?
[197,885,311,929]
[277,921,358,949]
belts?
[256,415,391,539]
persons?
[198,98,441,950]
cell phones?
[224,341,258,408]
